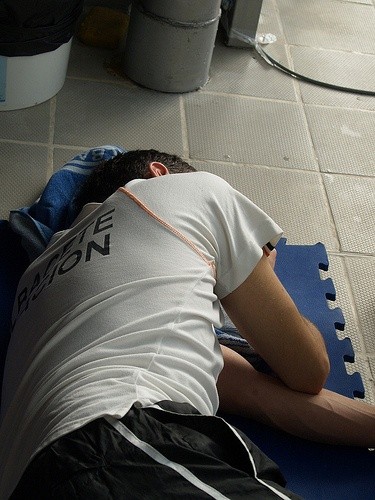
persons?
[0,142,375,500]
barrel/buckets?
[121,0,222,92]
[0,0,82,112]
